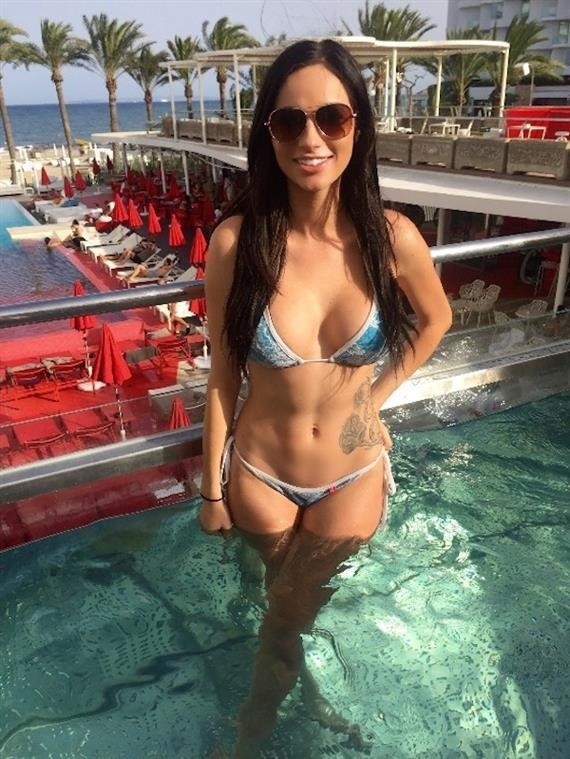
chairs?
[517,299,549,318]
[460,117,477,136]
[0,224,212,546]
[495,311,516,341]
[458,279,485,300]
[476,284,501,328]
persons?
[115,239,152,265]
[72,220,86,251]
[51,189,58,198]
[127,258,174,281]
[84,215,96,226]
[223,173,234,201]
[198,39,454,539]
[45,238,59,246]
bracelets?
[200,494,223,502]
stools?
[555,131,569,142]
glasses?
[264,103,359,142]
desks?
[506,124,546,140]
[428,121,461,136]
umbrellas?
[167,208,186,263]
[90,320,132,440]
[128,198,143,232]
[168,398,192,431]
[190,269,208,359]
[147,203,162,241]
[112,191,129,227]
[92,154,240,234]
[188,228,207,268]
[41,168,86,201]
[70,279,97,379]
